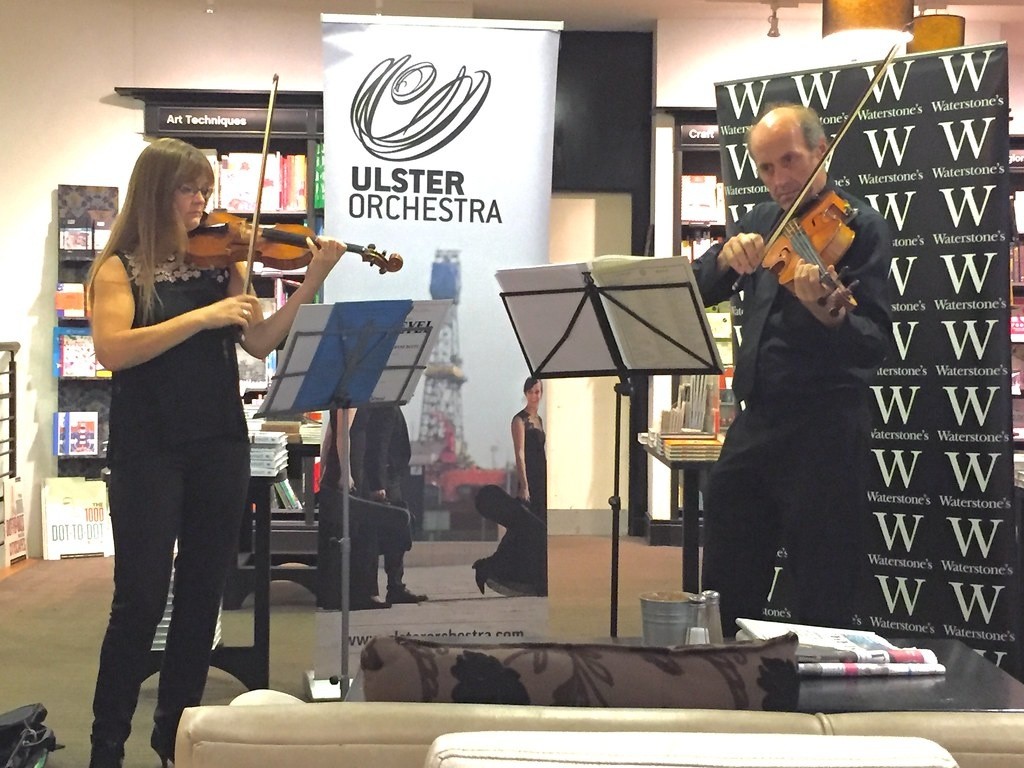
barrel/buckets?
[639,590,695,645]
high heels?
[150,726,175,768]
[89,737,126,768]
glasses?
[179,184,214,200]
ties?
[731,270,778,401]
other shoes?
[386,589,428,602]
[350,595,391,609]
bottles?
[683,590,724,646]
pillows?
[359,630,801,713]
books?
[53,183,119,456]
[638,374,722,462]
[198,148,326,509]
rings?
[242,309,248,319]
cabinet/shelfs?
[145,98,324,234]
[57,184,118,479]
[670,144,737,432]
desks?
[642,445,719,595]
[102,466,286,691]
[345,636,1024,712]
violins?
[762,190,861,317]
[177,209,403,275]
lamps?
[767,7,779,37]
[822,0,965,55]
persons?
[689,98,891,637]
[84,138,348,768]
[471,377,547,595]
[318,401,429,608]
